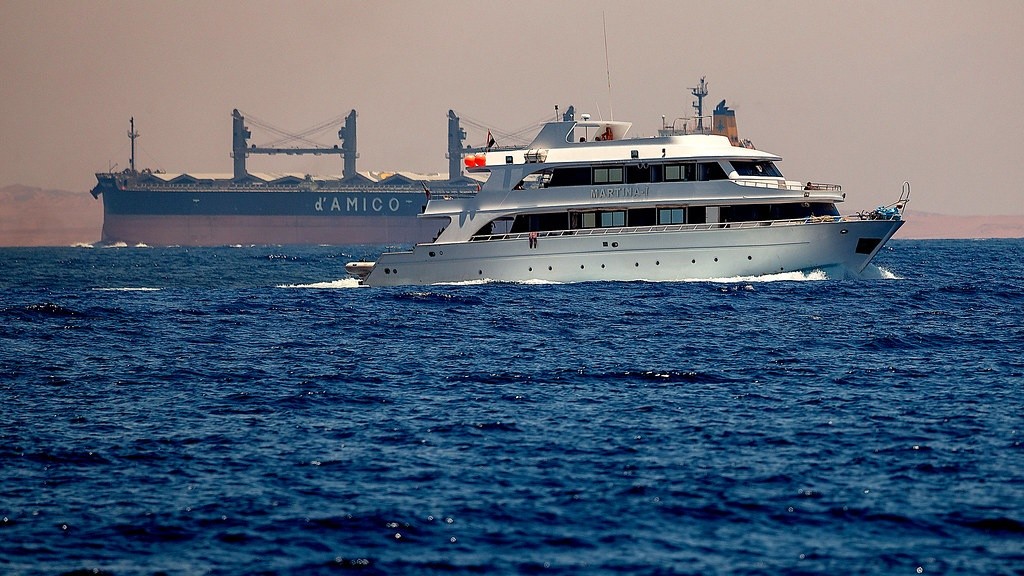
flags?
[486,129,497,152]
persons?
[481,147,485,152]
[803,182,814,197]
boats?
[345,76,913,287]
